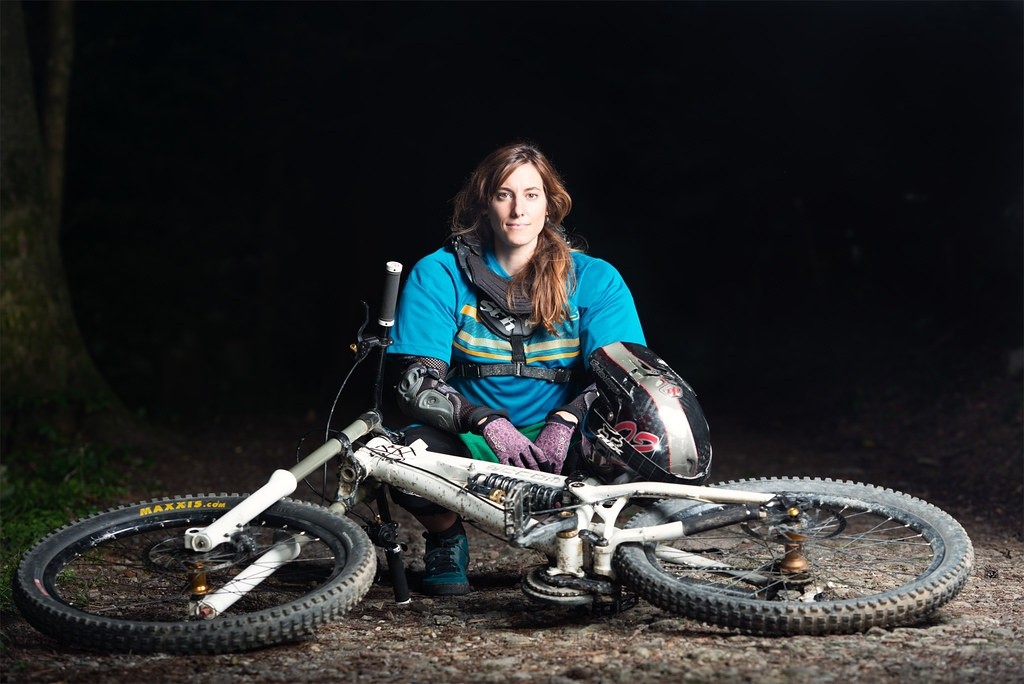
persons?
[383,144,647,595]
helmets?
[579,339,713,484]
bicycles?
[12,258,978,654]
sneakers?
[418,519,475,598]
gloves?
[533,412,577,476]
[482,413,551,474]
